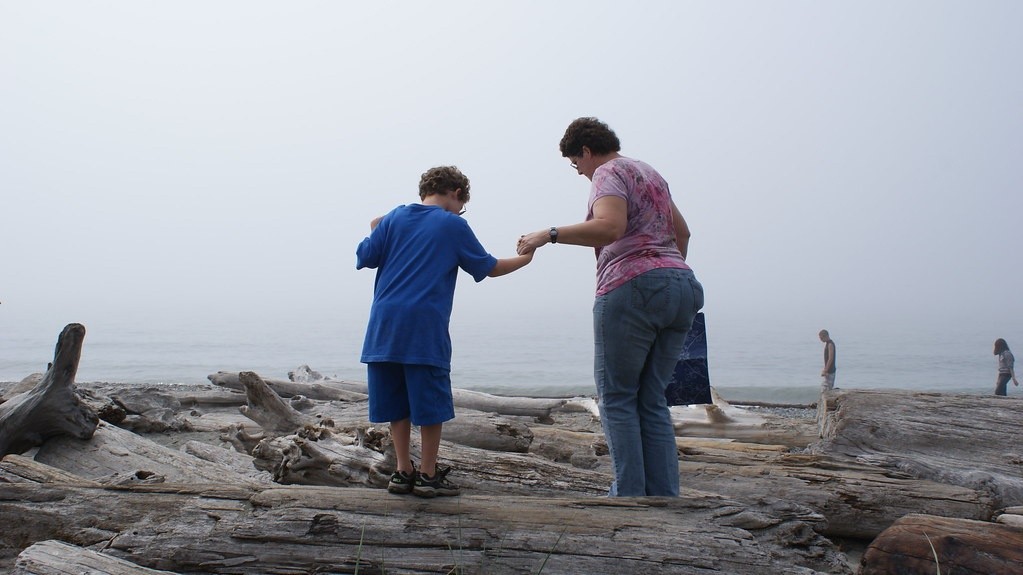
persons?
[517,116,704,496]
[819,330,835,390]
[994,338,1018,396]
[356,165,536,497]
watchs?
[550,226,557,244]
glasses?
[458,201,466,215]
[569,149,582,169]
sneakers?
[388,459,417,493]
[412,465,461,497]
